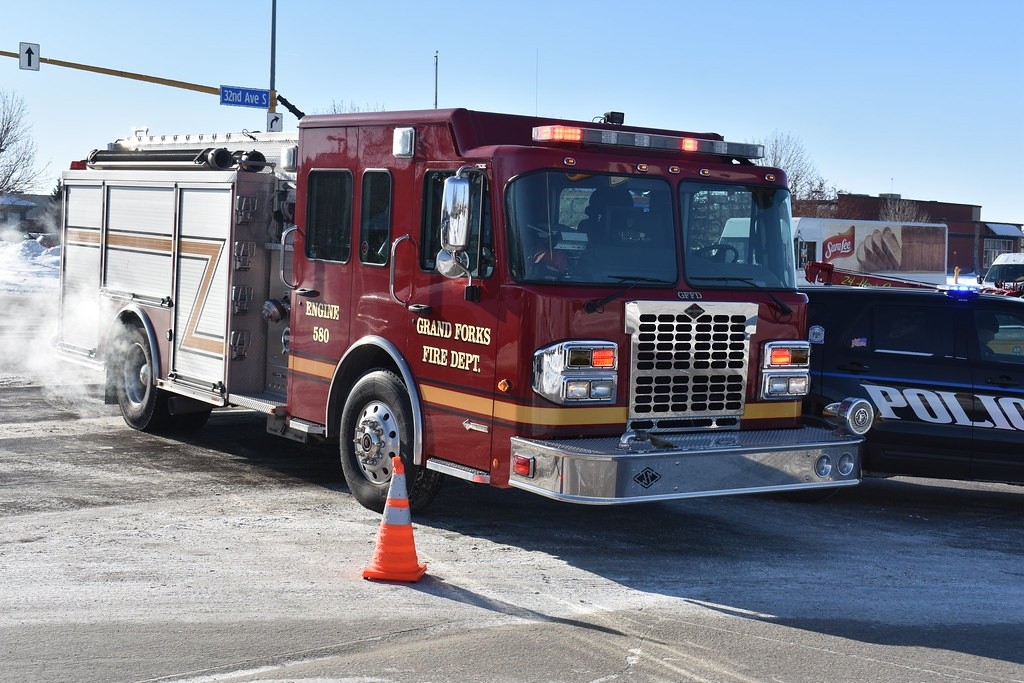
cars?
[793,250,1024,486]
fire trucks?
[50,106,879,518]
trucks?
[706,215,952,294]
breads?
[856,226,902,271]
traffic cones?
[362,458,431,583]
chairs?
[579,190,647,268]
